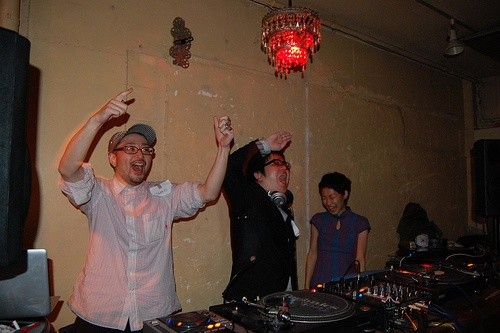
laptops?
[0,249,60,319]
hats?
[107,123,157,154]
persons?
[58,88,234,333]
[304,172,372,290]
[222,132,300,303]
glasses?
[263,159,292,169]
[113,145,155,155]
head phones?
[268,189,293,208]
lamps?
[261,0,321,75]
[443,18,465,55]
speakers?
[473,139,500,217]
